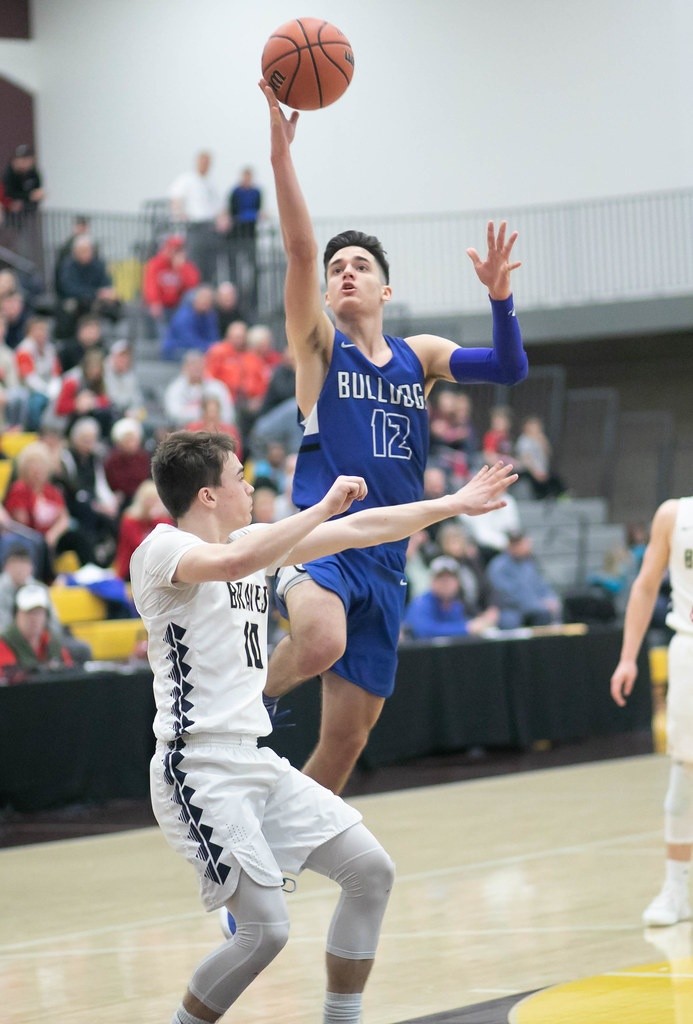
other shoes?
[258,702,295,748]
[219,907,236,939]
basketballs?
[260,16,355,112]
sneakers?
[644,921,692,955]
[645,888,691,926]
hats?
[14,585,48,613]
[429,556,458,580]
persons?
[0,148,674,754]
[128,429,517,1024]
[612,493,693,928]
[221,78,530,939]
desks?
[0,625,656,811]
